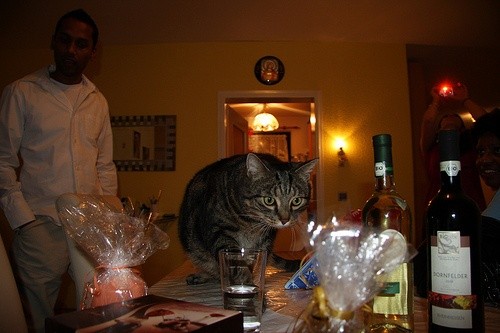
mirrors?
[111,115,176,171]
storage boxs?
[45,294,244,333]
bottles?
[426,129,485,333]
[361,133,414,333]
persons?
[420,77,500,306]
[0,9,119,333]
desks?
[146,258,500,333]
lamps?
[332,137,347,155]
[437,75,454,97]
[251,103,279,131]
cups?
[218,248,268,333]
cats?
[178,152,321,285]
